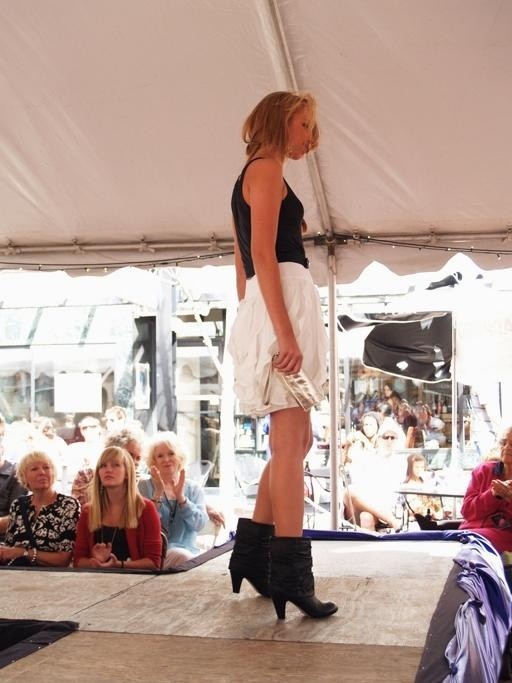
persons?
[337,381,450,534]
[222,88,340,621]
[457,424,511,555]
[1,403,226,577]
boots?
[267,536,338,619]
[229,517,275,598]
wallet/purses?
[262,349,326,411]
